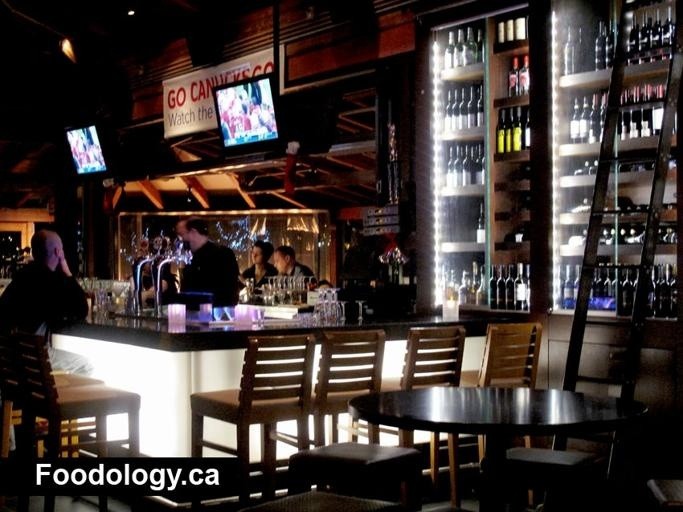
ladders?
[545,0,682,487]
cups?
[200,302,265,325]
[167,303,188,326]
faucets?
[154,236,193,316]
[134,237,172,316]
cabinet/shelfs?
[414,0,536,322]
[539,0,680,348]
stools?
[284,444,425,511]
[480,447,598,508]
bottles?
[561,5,683,318]
[435,24,488,307]
[494,10,530,153]
[489,259,530,314]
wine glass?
[262,275,317,307]
[309,286,345,329]
[76,276,131,316]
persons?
[273,246,314,277]
[175,216,239,311]
[0,230,93,375]
[133,258,177,307]
[242,239,279,294]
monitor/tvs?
[65,125,108,175]
[211,73,280,148]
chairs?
[0,333,141,512]
[461,322,542,509]
[310,330,386,449]
[190,335,315,509]
[384,326,466,509]
